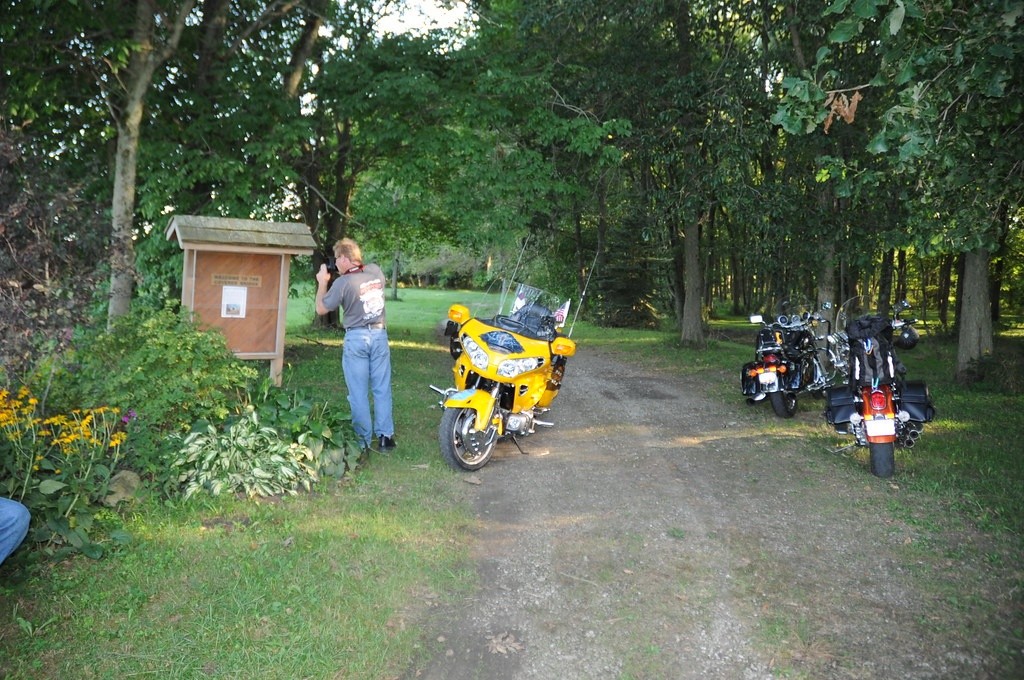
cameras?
[326,259,339,273]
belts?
[346,323,386,329]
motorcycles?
[429,275,576,471]
[814,294,936,479]
[740,293,837,421]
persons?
[315,238,397,452]
[1,497,31,566]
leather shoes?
[378,434,396,451]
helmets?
[893,325,920,350]
